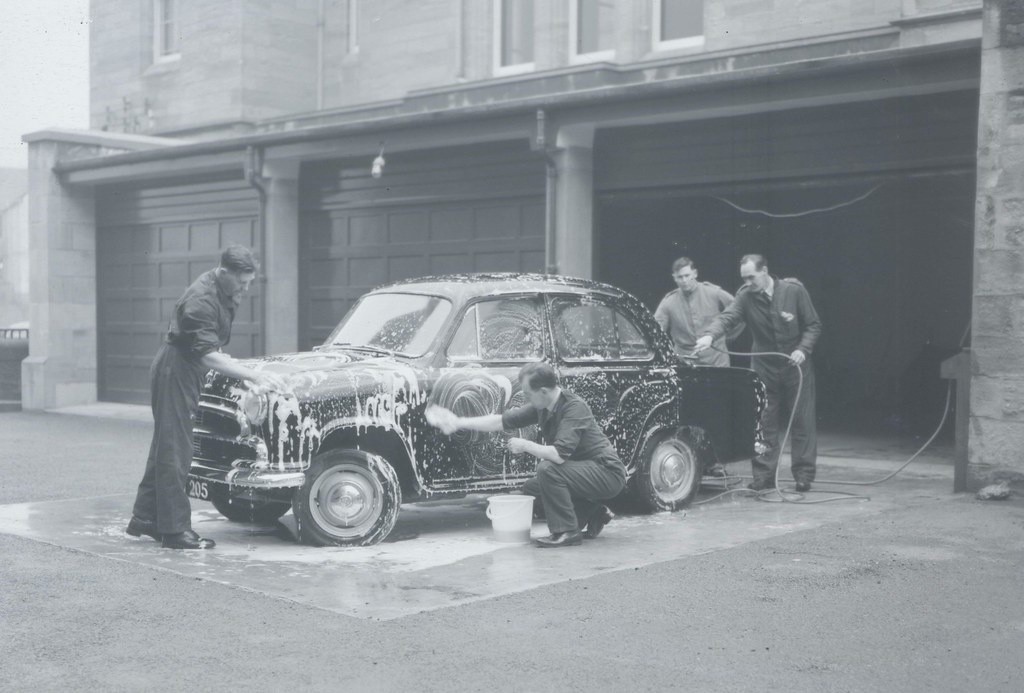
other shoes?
[740,481,776,496]
[702,466,727,477]
[796,481,811,491]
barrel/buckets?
[486,495,536,542]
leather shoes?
[536,528,582,547]
[126,516,162,543]
[581,504,616,539]
[161,529,216,550]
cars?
[182,272,770,547]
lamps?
[369,143,387,180]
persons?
[434,361,627,547]
[651,257,747,476]
[694,253,822,492]
[128,244,289,549]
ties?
[762,292,771,304]
[540,408,548,426]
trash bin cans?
[0,329,29,412]
